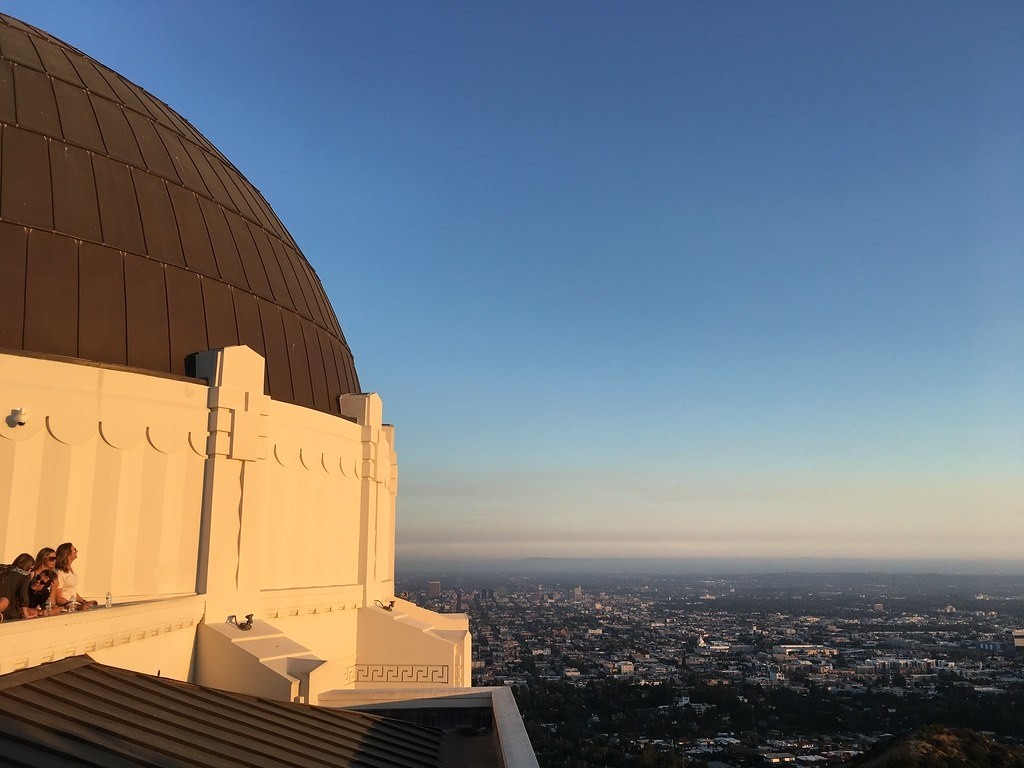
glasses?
[48,557,59,562]
[35,575,48,585]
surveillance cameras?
[15,414,28,426]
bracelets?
[81,600,86,604]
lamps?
[375,599,395,611]
[230,614,253,631]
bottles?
[45,597,52,616]
[106,592,112,607]
[69,595,76,612]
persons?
[0,547,65,624]
[56,543,98,610]
